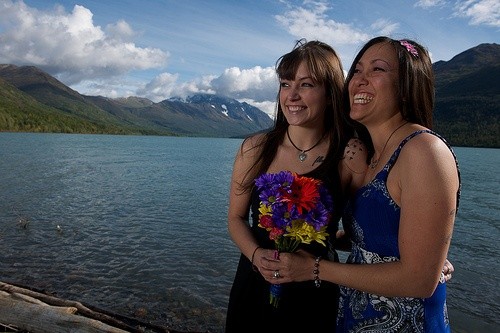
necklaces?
[368,123,406,169]
[285,127,325,161]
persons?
[225,40,454,333]
[257,36,462,333]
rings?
[272,271,280,280]
[446,264,451,274]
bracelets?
[251,246,263,267]
[313,256,323,287]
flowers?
[254,171,333,309]
[402,40,419,58]
